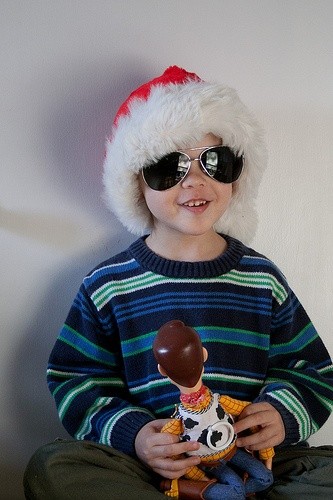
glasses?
[139,145,245,193]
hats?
[103,65,267,241]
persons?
[20,63,333,500]
[152,322,275,499]
